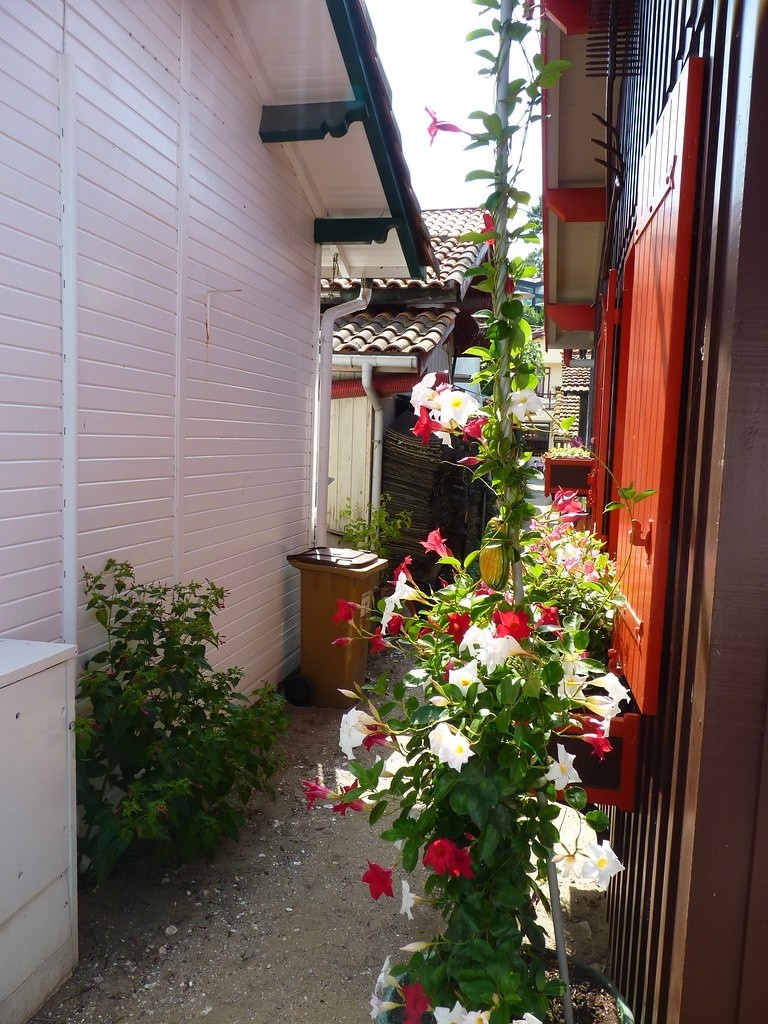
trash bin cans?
[286,544,388,709]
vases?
[373,939,637,1024]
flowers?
[288,108,621,1024]
